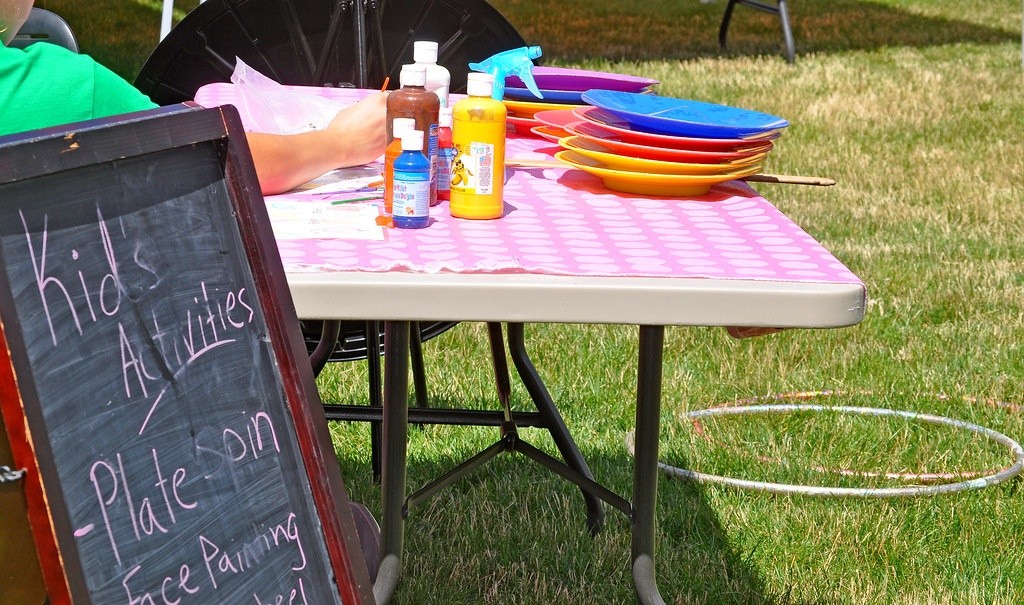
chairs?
[130,0,542,490]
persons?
[0,1,388,196]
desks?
[189,83,868,604]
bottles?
[400,41,451,122]
[435,107,452,201]
[385,119,416,213]
[449,72,507,220]
[392,130,431,228]
[385,65,440,206]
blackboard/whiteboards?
[1,106,376,605]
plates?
[502,66,789,197]
